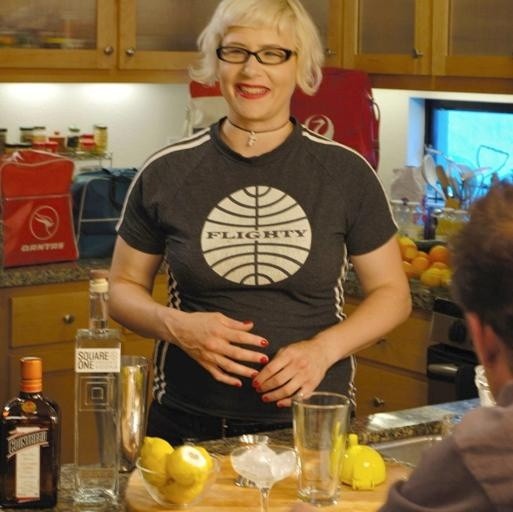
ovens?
[425,342,486,410]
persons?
[373,172,512,512]
[103,1,413,472]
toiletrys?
[394,197,469,239]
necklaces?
[226,116,289,147]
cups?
[290,390,351,507]
[92,351,150,477]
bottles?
[2,353,61,508]
[0,123,108,157]
[71,269,120,506]
[394,197,469,242]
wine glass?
[237,434,268,487]
[229,443,298,512]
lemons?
[140,437,212,505]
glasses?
[215,44,299,66]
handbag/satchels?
[0,147,79,271]
[71,166,142,259]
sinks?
[371,435,443,465]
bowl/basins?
[134,448,225,511]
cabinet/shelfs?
[0,271,170,466]
[296,0,342,68]
[342,1,431,76]
[0,1,222,71]
[431,1,513,79]
[340,293,432,420]
